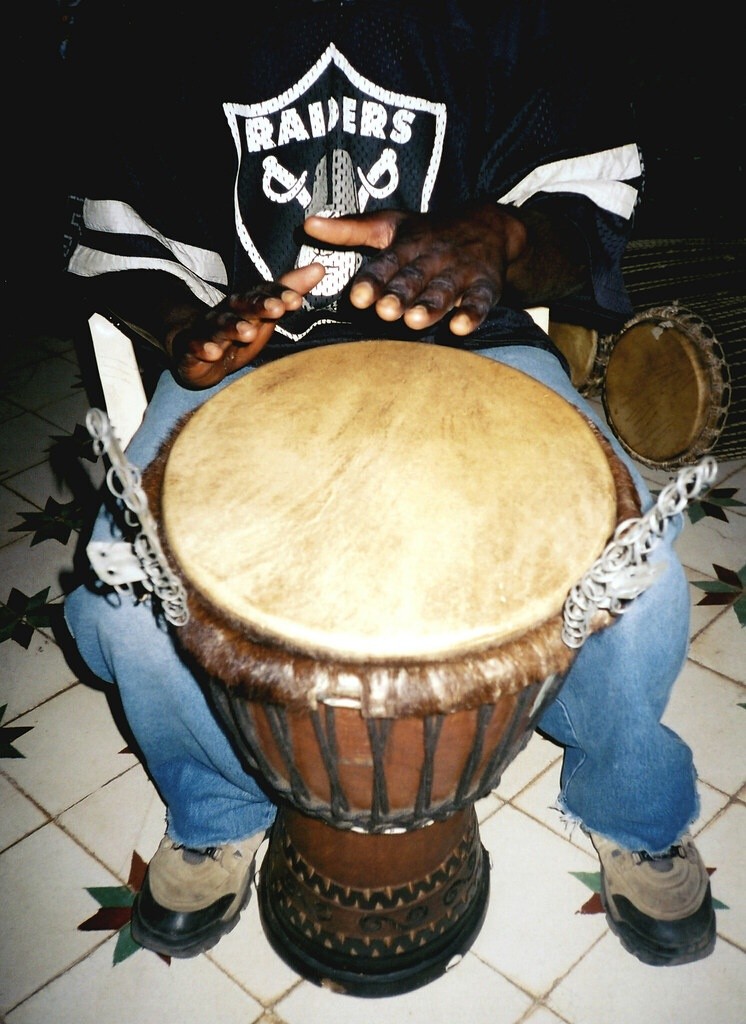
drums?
[75,335,721,1006]
[545,231,746,474]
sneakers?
[588,825,718,967]
[129,826,269,958]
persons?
[60,2,719,964]
